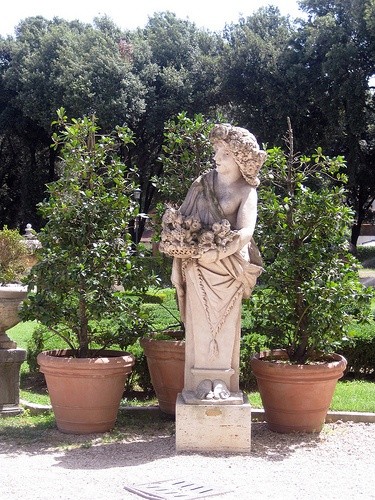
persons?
[171,123,267,400]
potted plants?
[0,225,35,350]
[17,107,375,434]
